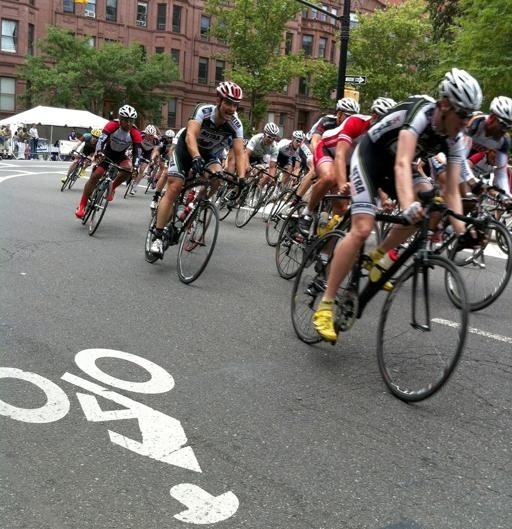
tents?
[0,105,111,161]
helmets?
[216,81,243,104]
[145,124,156,136]
[335,97,360,114]
[264,122,279,136]
[91,128,101,138]
[489,95,512,125]
[293,130,305,141]
[165,130,175,137]
[118,104,137,120]
[438,67,481,111]
[370,96,397,117]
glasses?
[92,136,99,139]
[295,139,302,143]
[265,133,276,141]
[456,111,472,121]
[498,121,512,131]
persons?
[23,128,30,144]
[146,129,175,188]
[212,147,228,169]
[225,122,280,202]
[282,97,361,218]
[467,150,498,179]
[312,67,483,342]
[256,129,306,198]
[389,156,444,262]
[61,127,102,182]
[15,127,22,143]
[29,123,39,160]
[76,103,142,218]
[122,124,157,195]
[243,138,249,151]
[51,140,59,160]
[3,123,12,159]
[68,130,79,141]
[150,81,246,255]
[426,94,512,251]
[298,96,398,234]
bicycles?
[264,165,300,221]
[266,190,302,246]
[124,155,148,201]
[81,154,137,237]
[217,160,280,228]
[144,164,249,284]
[482,194,511,254]
[59,149,93,192]
[276,193,363,281]
[444,185,511,313]
[290,188,490,402]
[144,157,167,194]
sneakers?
[297,213,311,237]
[150,193,160,210]
[224,190,236,202]
[106,190,115,201]
[61,176,68,184]
[388,248,399,261]
[75,205,85,219]
[362,251,394,292]
[311,299,337,343]
[150,231,163,258]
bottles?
[180,201,195,222]
[379,250,397,270]
[316,211,328,234]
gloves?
[192,155,205,174]
